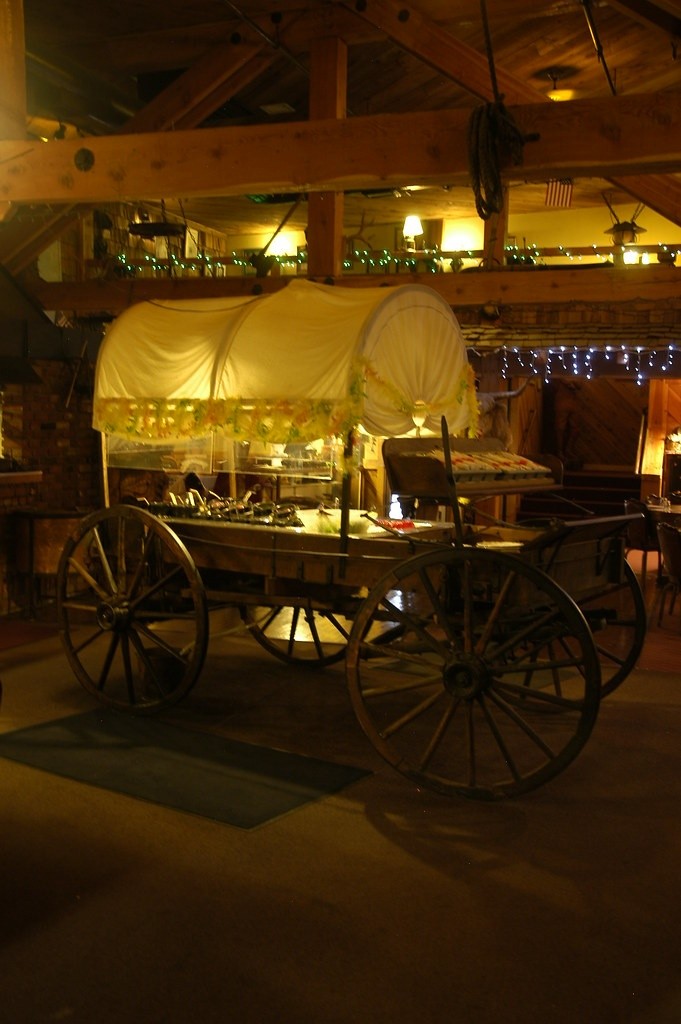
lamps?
[603,191,646,246]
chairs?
[624,499,662,594]
[654,522,681,625]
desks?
[645,504,681,526]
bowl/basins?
[317,508,378,534]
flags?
[544,175,575,208]
